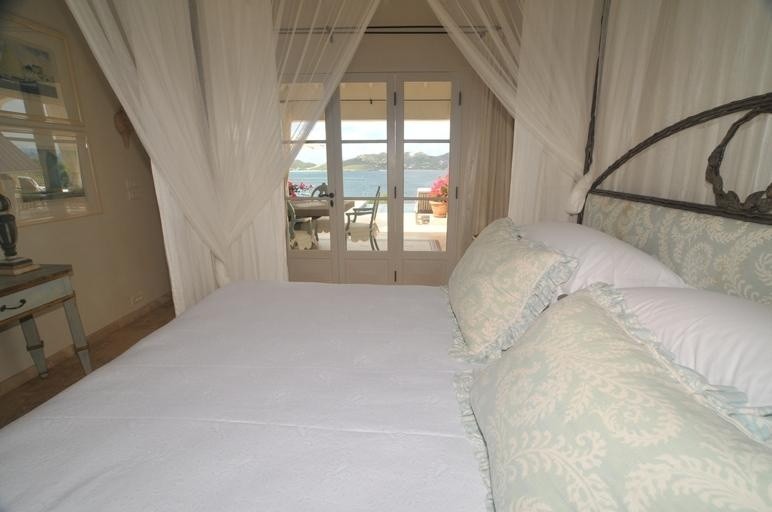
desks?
[0,263,98,381]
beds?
[0,91,771,512]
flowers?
[427,175,449,202]
[288,180,314,203]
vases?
[428,201,447,218]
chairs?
[286,183,382,254]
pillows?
[518,219,691,292]
[594,279,771,443]
[461,281,771,512]
[447,217,570,363]
